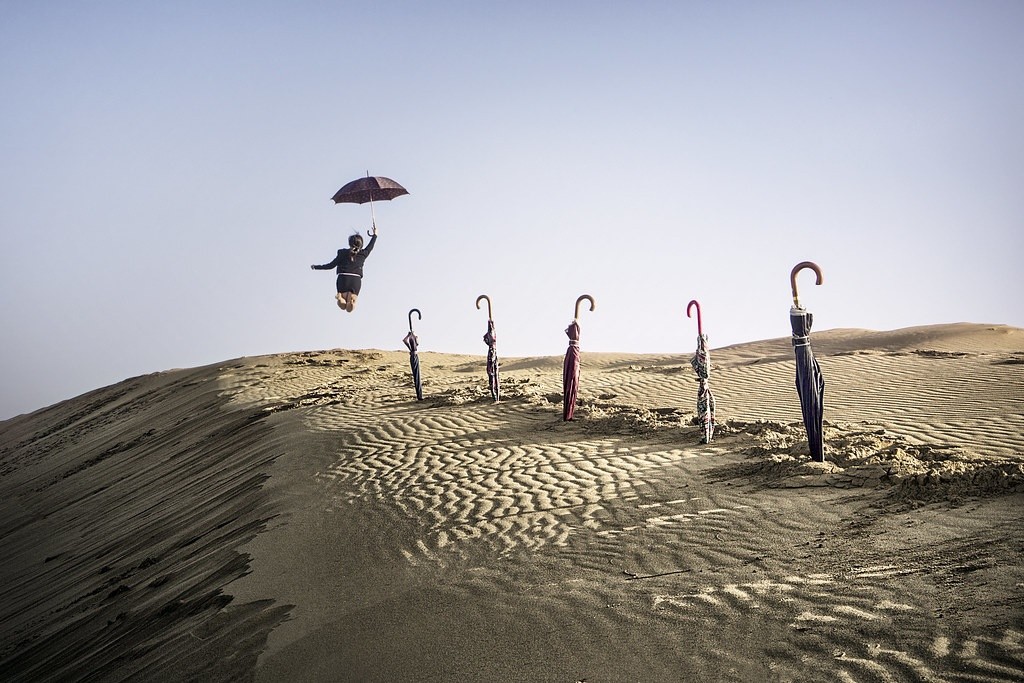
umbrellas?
[687,300,715,444]
[790,261,825,462]
[402,309,422,400]
[330,170,410,236]
[563,294,595,421]
[476,295,500,401]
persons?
[311,228,378,313]
